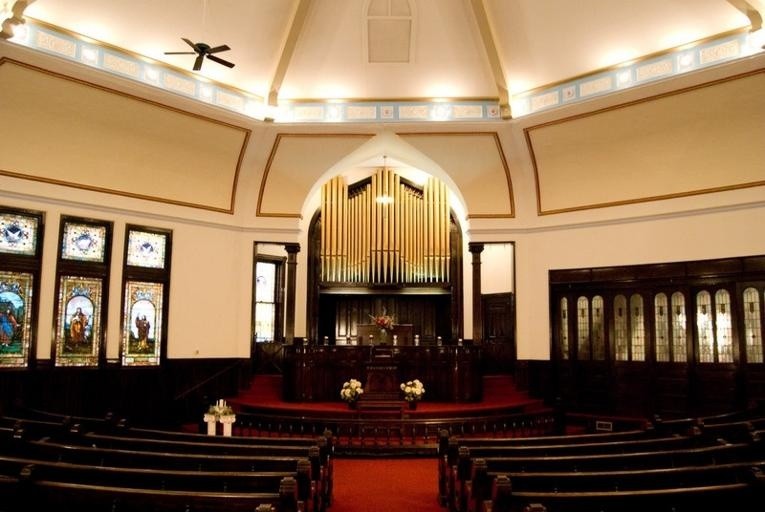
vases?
[380,328,388,344]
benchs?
[435,401,765,511]
[0,400,337,511]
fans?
[164,36,235,72]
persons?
[69,307,89,348]
[0,303,20,348]
[135,310,150,351]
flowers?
[399,378,426,402]
[340,378,365,404]
[368,305,401,331]
